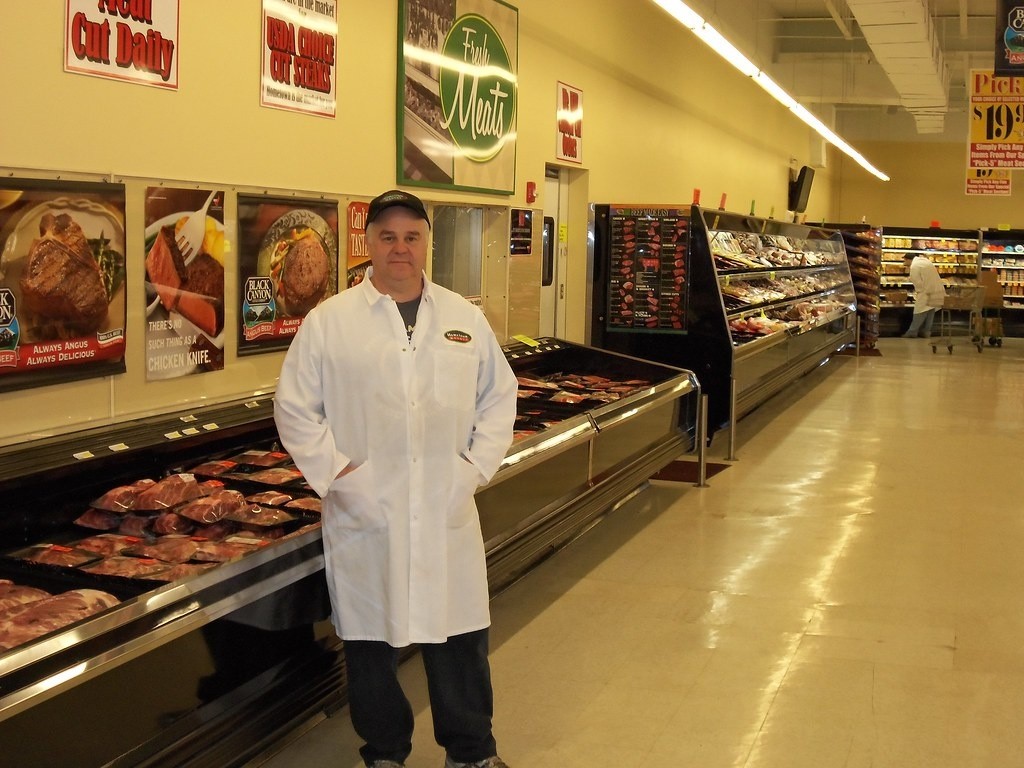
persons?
[901,253,949,338]
[273,188,518,768]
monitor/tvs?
[791,165,815,212]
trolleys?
[929,286,1005,354]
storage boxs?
[981,271,1003,309]
[975,318,1004,336]
[886,291,908,302]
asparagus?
[85,229,117,302]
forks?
[174,191,216,265]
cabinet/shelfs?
[718,260,850,320]
[881,247,978,287]
[982,250,1024,300]
[841,231,882,348]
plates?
[257,210,337,323]
[0,198,125,362]
[144,212,226,381]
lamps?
[652,0,890,182]
